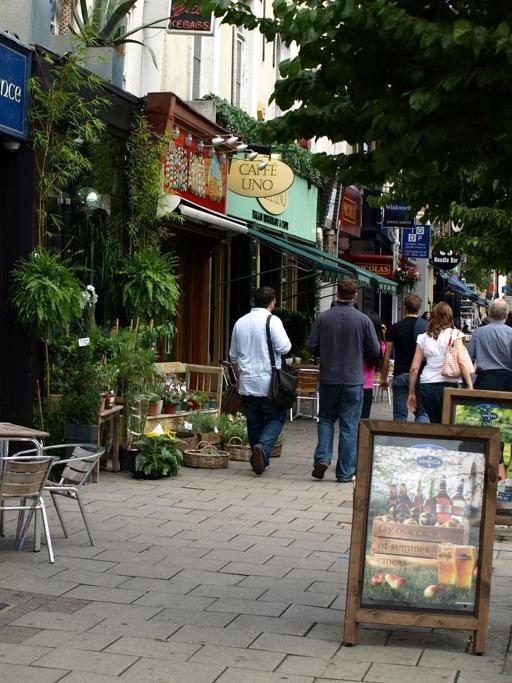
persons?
[229,287,292,476]
[307,278,384,482]
[468,299,512,392]
[407,302,476,422]
[379,293,430,422]
[360,312,387,419]
[462,320,469,333]
[421,311,430,319]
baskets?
[223,437,253,462]
[184,441,230,468]
[270,446,282,457]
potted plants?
[60,386,99,444]
[68,0,178,85]
[78,324,164,416]
[271,308,312,365]
[188,391,209,410]
[170,412,225,451]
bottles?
[386,484,397,523]
[450,481,465,516]
[395,484,410,522]
[431,476,450,525]
[410,480,423,523]
[498,441,506,479]
[421,481,436,524]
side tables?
[92,405,124,483]
[286,363,320,419]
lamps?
[258,161,269,170]
[227,134,239,146]
[247,149,259,161]
[212,135,225,145]
[236,144,249,152]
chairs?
[0,443,106,551]
[289,367,321,423]
[108,361,224,451]
[0,455,61,564]
[375,359,394,406]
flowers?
[131,425,184,476]
[163,375,188,405]
[221,412,284,446]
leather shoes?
[312,459,329,480]
[337,475,355,482]
[251,443,267,476]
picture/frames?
[440,388,511,531]
[342,420,503,656]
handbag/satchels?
[266,313,300,411]
[441,326,476,378]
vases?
[164,405,176,414]
[174,404,181,411]
[223,442,283,458]
[133,471,161,480]
[181,403,188,411]
